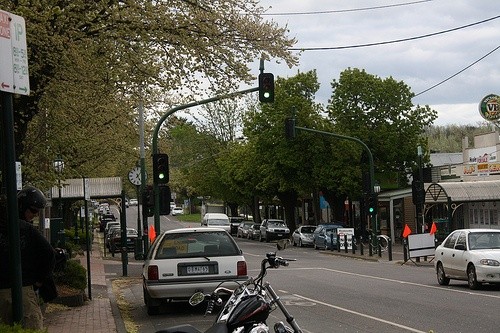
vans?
[200,212,231,234]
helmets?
[16,185,46,220]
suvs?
[259,219,290,242]
[228,217,244,236]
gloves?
[55,248,67,262]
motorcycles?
[188,251,304,333]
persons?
[0,188,57,332]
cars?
[434,228,500,290]
[98,199,138,253]
[237,221,255,238]
[247,224,260,240]
[141,227,249,316]
[292,225,317,247]
[313,224,357,250]
[172,207,184,216]
[170,202,176,210]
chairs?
[162,248,177,256]
[204,244,217,252]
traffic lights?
[154,153,169,184]
[258,73,274,103]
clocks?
[127,166,148,186]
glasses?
[28,207,43,215]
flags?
[430,222,438,236]
[403,224,411,238]
